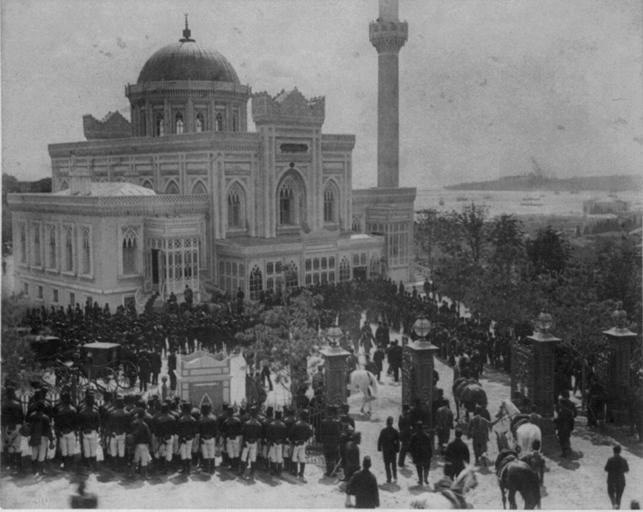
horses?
[495,430,542,510]
[495,396,542,455]
[453,376,488,421]
[307,356,380,416]
[409,460,479,509]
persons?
[1,273,628,510]
